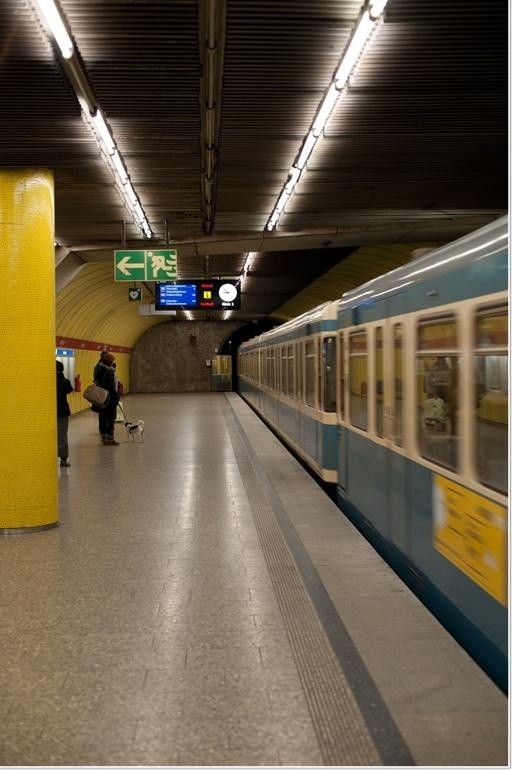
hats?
[101,353,116,365]
[57,361,64,371]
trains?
[236,214,508,695]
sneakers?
[103,441,119,446]
[60,463,71,467]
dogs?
[124,420,145,443]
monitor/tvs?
[154,281,199,309]
[198,280,241,311]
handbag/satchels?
[115,401,124,423]
[83,383,110,408]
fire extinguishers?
[75,374,81,392]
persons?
[424,356,484,434]
[90,352,120,446]
[56,358,73,466]
